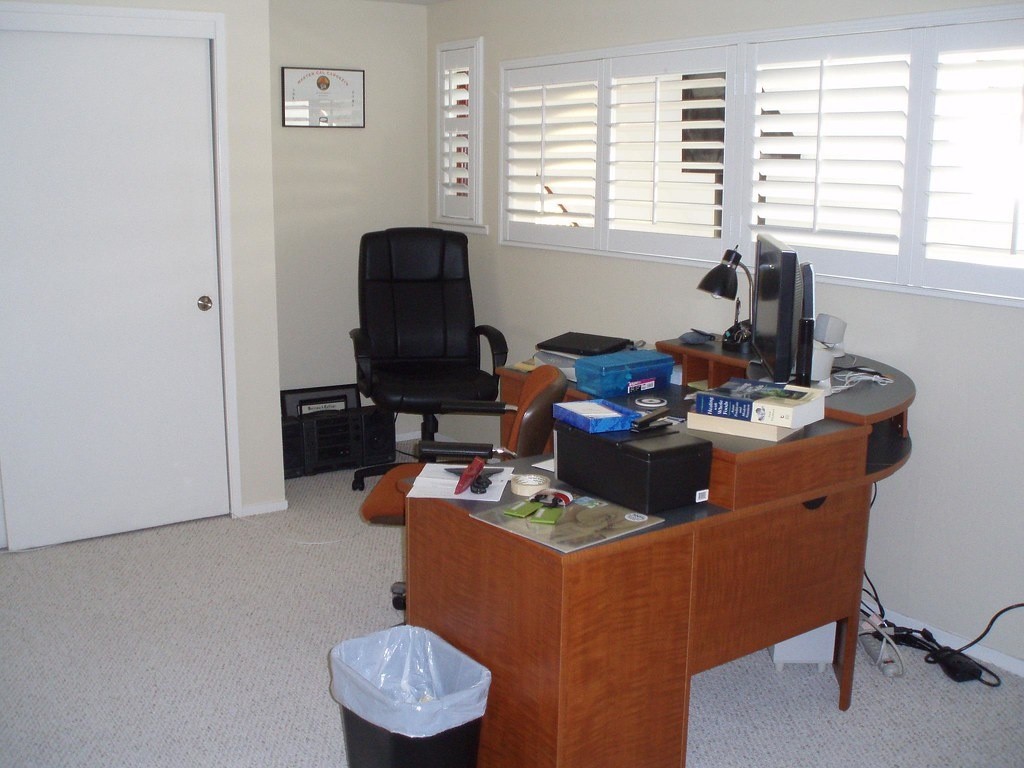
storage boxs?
[552,399,642,434]
[554,421,714,515]
[297,404,397,475]
[573,349,676,399]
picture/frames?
[281,66,365,129]
[280,383,362,420]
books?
[686,375,827,442]
[468,487,666,554]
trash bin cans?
[328,625,492,768]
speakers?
[814,313,847,357]
[810,347,835,396]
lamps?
[697,244,754,355]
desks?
[403,338,917,768]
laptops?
[537,332,630,357]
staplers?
[631,407,673,433]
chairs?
[358,364,570,610]
[349,226,510,490]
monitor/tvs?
[751,232,816,388]
